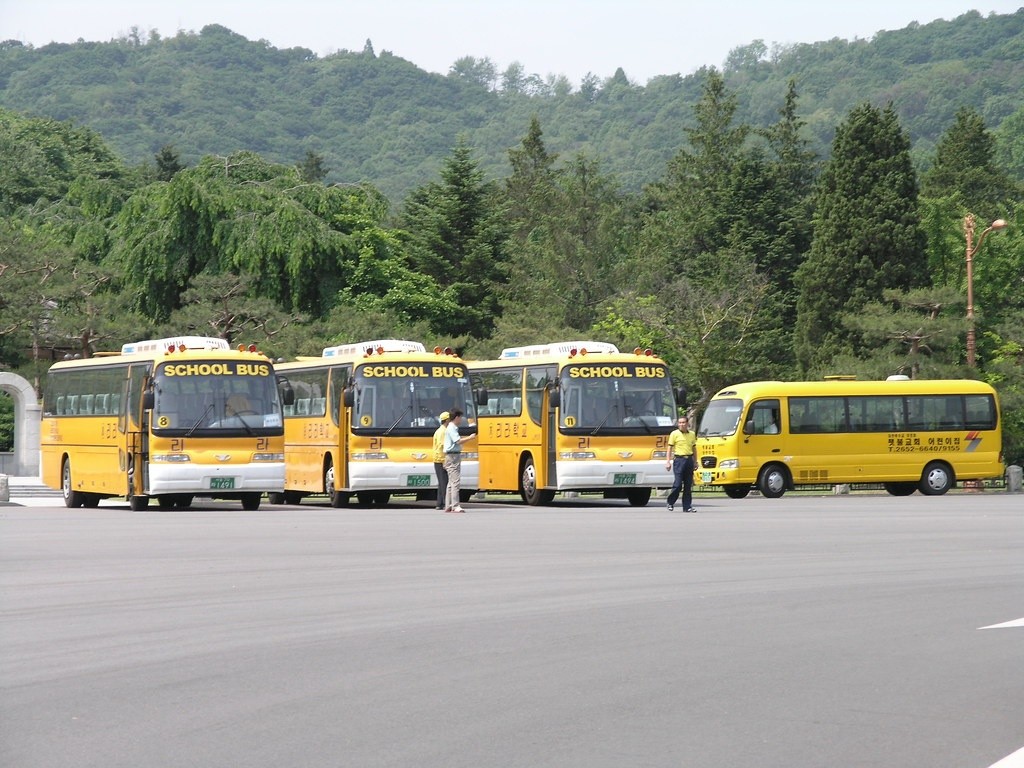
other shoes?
[436,506,445,511]
[683,506,697,514]
[667,504,674,512]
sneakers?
[451,504,464,513]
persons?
[432,411,451,510]
[665,415,697,513]
[442,407,476,513]
[764,413,778,434]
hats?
[440,412,450,421]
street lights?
[964,214,1009,374]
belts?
[675,456,693,458]
[446,452,461,455]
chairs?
[54,382,995,437]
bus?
[39,334,286,512]
[260,338,488,507]
[455,340,686,506]
[691,372,1004,497]
